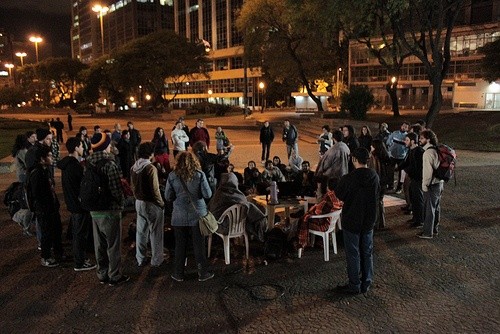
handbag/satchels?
[198,211,218,236]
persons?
[3,111,445,286]
[334,146,381,294]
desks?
[253,195,308,230]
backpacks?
[3,182,32,231]
[427,144,457,184]
[79,159,114,210]
[262,227,291,265]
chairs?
[207,204,249,265]
[298,209,342,261]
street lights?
[29,36,42,65]
[92,4,109,55]
[0,63,14,88]
[15,52,27,67]
[259,82,264,113]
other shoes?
[99,275,128,286]
[361,279,369,294]
[149,261,168,278]
[171,274,183,282]
[401,205,413,215]
[198,271,214,281]
[74,258,97,271]
[41,258,66,267]
[385,188,395,195]
[415,231,439,239]
[352,287,361,295]
[135,257,151,269]
[393,189,402,194]
[289,213,301,218]
[407,218,424,228]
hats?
[380,123,388,128]
[36,128,51,141]
[91,132,110,153]
[349,146,371,159]
[411,119,426,129]
